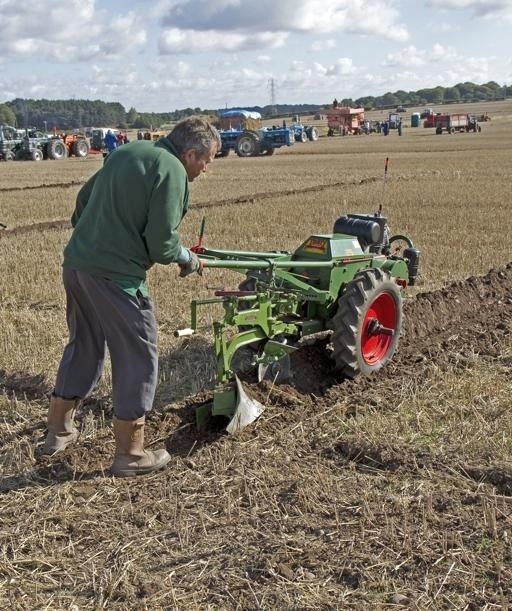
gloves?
[178,248,200,277]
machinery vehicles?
[421,108,491,135]
[214,120,319,158]
[353,113,403,136]
[396,105,406,112]
[0,125,119,161]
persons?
[381,121,389,137]
[42,114,224,478]
[397,121,403,137]
[333,98,338,109]
[137,130,143,140]
[103,129,119,154]
[144,129,151,140]
[117,131,124,146]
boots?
[43,392,80,455]
[110,414,171,476]
